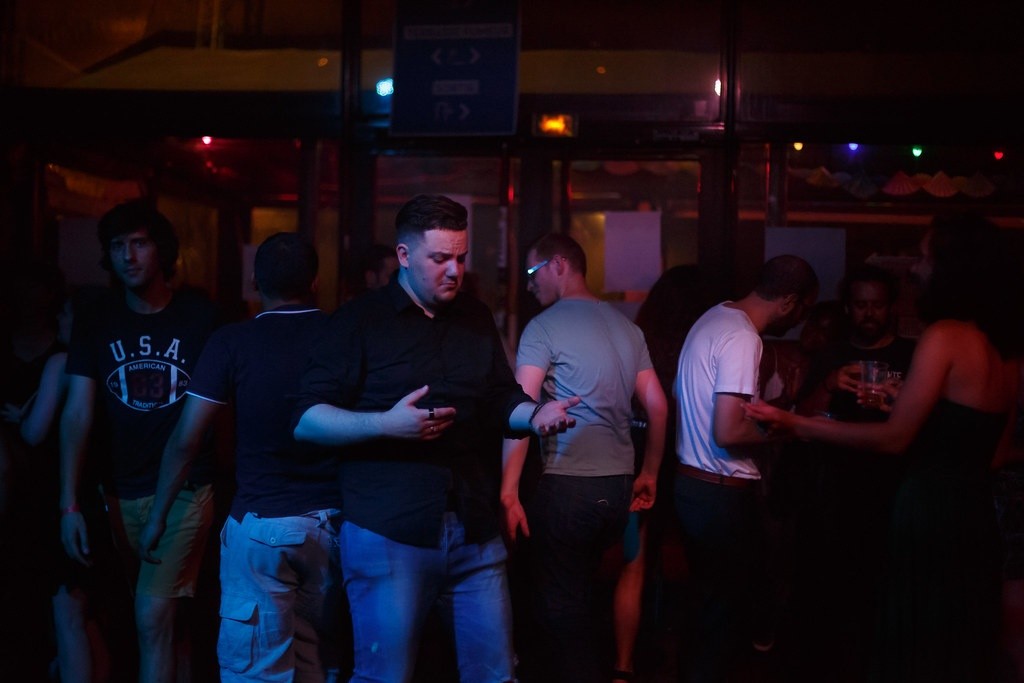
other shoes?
[752,632,775,651]
[608,669,638,683]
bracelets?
[60,506,81,514]
[529,401,551,432]
[824,370,838,393]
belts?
[679,464,761,491]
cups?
[862,361,890,407]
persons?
[0,192,1024,683]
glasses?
[526,254,569,277]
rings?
[429,408,435,421]
[430,426,434,434]
